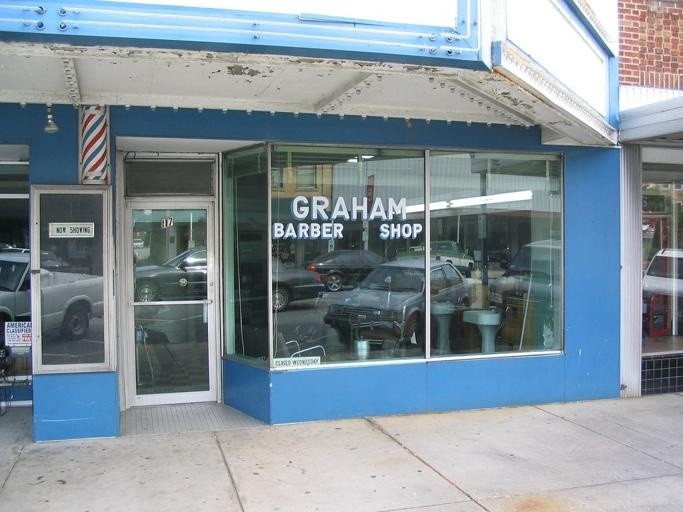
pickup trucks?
[0,247,105,358]
[396,239,475,277]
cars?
[642,246,682,322]
[490,237,562,317]
[135,247,207,302]
[305,249,395,293]
[325,258,483,344]
[242,254,322,313]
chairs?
[133,316,193,389]
[273,330,327,361]
